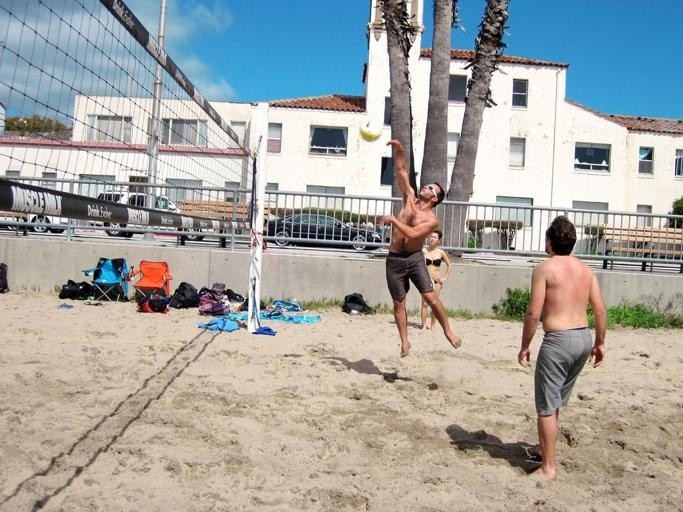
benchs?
[179,200,270,249]
[603,224,683,273]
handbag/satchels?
[59,280,95,300]
[169,282,200,309]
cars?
[0,211,69,234]
[266,211,382,252]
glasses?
[425,184,437,198]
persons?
[386,140,461,357]
[419,230,452,331]
[517,215,606,481]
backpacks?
[341,292,376,316]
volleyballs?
[360,119,382,141]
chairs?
[81,257,129,301]
[131,261,171,299]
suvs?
[89,188,204,241]
